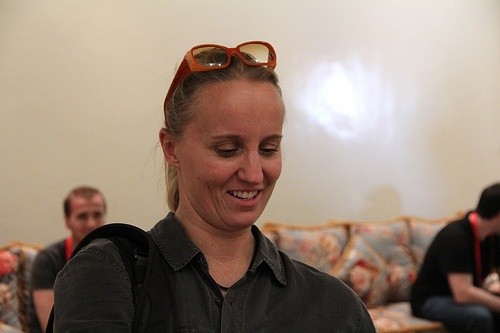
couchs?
[261,212,471,333]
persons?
[27,185,107,333]
[53,41,377,333]
[411,182,500,333]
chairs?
[0,241,42,333]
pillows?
[330,234,389,309]
[388,241,418,303]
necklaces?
[66,237,74,262]
[470,212,482,284]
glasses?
[164,40,276,114]
